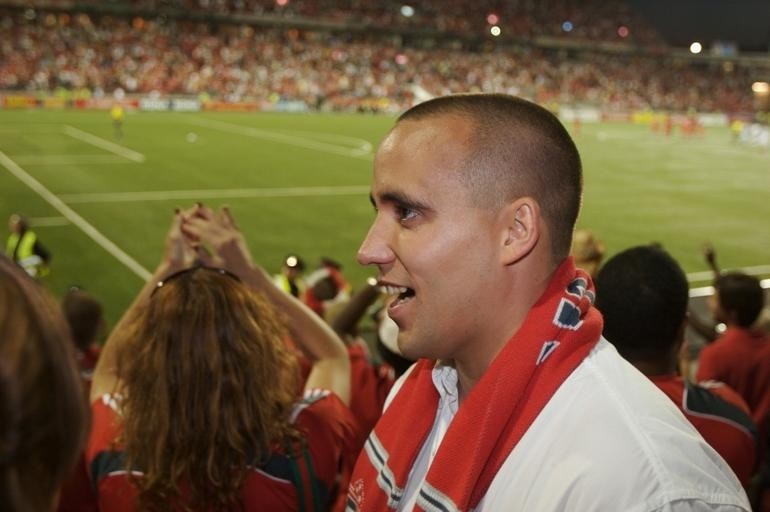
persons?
[569,225,770,508]
[335,92,754,510]
[85,199,353,511]
[63,293,101,394]
[270,255,416,435]
[4,214,53,280]
